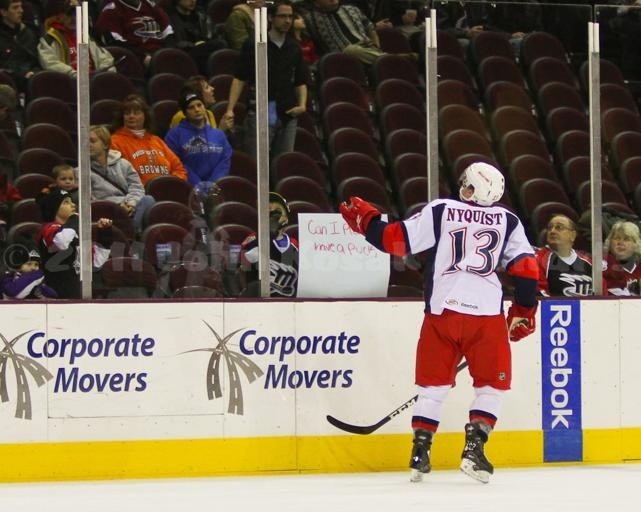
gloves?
[506,301,538,342]
[270,209,284,240]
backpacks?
[577,205,641,251]
[186,37,228,55]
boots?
[408,430,434,474]
[461,422,495,474]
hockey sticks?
[327,321,526,435]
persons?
[338,161,541,474]
[603,219,641,296]
[534,212,593,296]
[1,2,531,301]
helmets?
[457,161,506,206]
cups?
[229,245,241,265]
[156,242,171,268]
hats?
[36,189,69,221]
[16,247,41,265]
[63,0,82,12]
[177,91,205,116]
[341,195,381,235]
[269,191,291,214]
